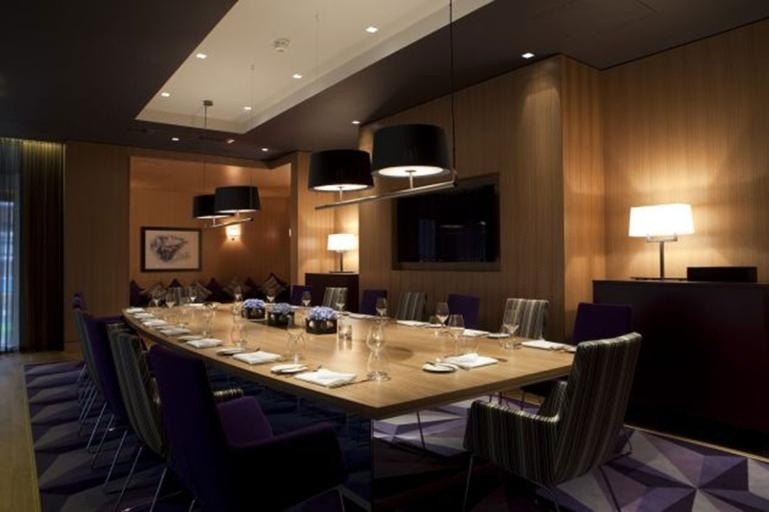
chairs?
[462,331,642,510]
[288,284,315,307]
[359,288,388,317]
[70,291,243,511]
[490,296,551,411]
[394,291,428,321]
[148,342,352,509]
[321,286,349,312]
[441,293,482,330]
[519,300,635,414]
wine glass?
[152,285,522,379]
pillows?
[222,272,251,303]
[256,272,291,303]
[130,279,153,306]
[205,277,236,303]
[185,279,213,303]
[246,276,268,301]
[168,278,183,289]
[146,282,172,307]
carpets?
[23,361,769,512]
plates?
[126,302,511,374]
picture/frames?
[139,226,203,273]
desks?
[120,301,579,511]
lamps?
[192,100,261,234]
[308,0,458,212]
[327,232,356,273]
[627,201,694,278]
